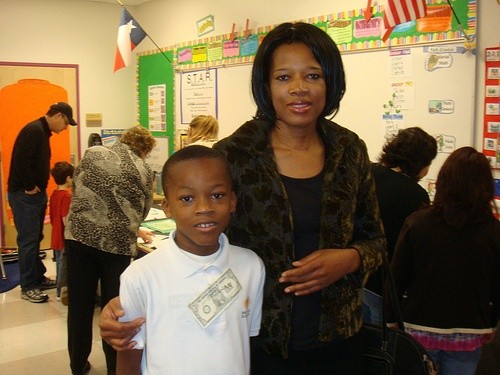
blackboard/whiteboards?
[173,38,477,204]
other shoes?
[61,287,69,306]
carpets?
[0,262,19,293]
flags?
[113,7,148,72]
[381,0,428,43]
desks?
[134,194,175,260]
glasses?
[61,114,69,126]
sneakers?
[37,278,58,291]
[21,288,48,303]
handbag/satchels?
[354,243,424,375]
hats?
[52,102,78,126]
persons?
[7,102,77,303]
[370,128,438,326]
[116,144,267,375]
[98,22,387,375]
[188,116,218,148]
[50,133,153,305]
[65,127,155,375]
[390,147,500,375]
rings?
[145,240,148,243]
